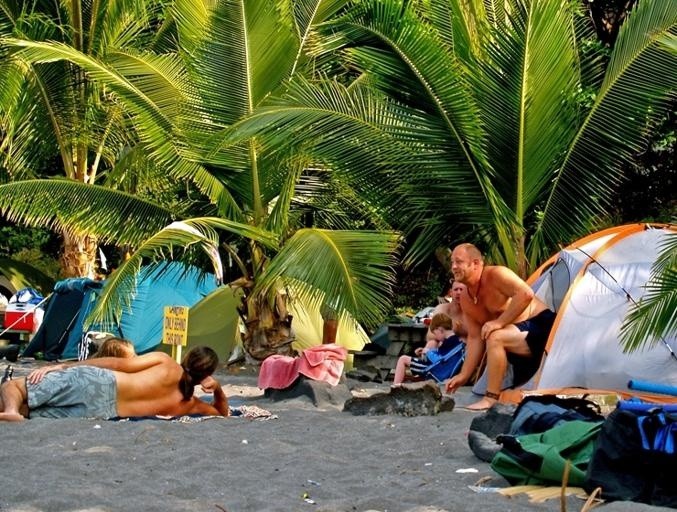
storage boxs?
[4,303,36,331]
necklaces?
[469,270,483,305]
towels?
[255,341,348,391]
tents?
[158,275,371,375]
[470,224,676,405]
[18,262,223,361]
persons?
[424,279,468,349]
[394,312,464,384]
[444,242,556,411]
[1,345,230,422]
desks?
[0,329,32,354]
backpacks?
[490,419,605,487]
[581,397,677,509]
[505,393,606,435]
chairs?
[422,343,467,383]
